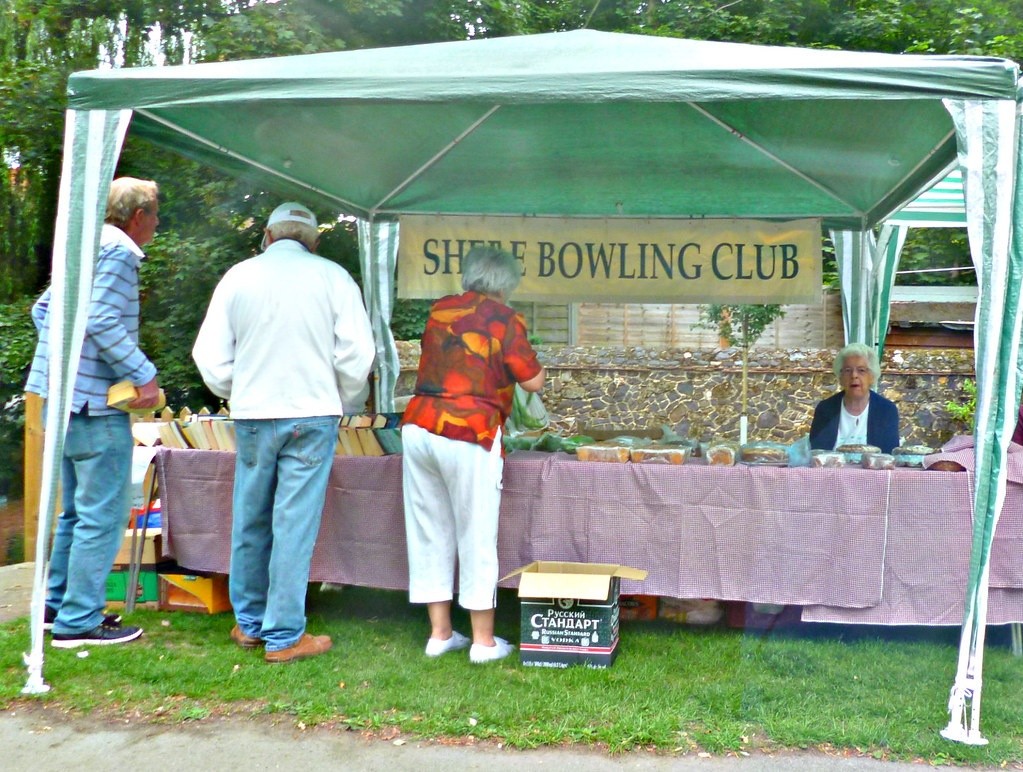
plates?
[740,461,789,467]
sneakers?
[425,630,471,656]
[471,636,517,663]
[44,607,121,630]
[51,627,142,648]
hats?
[261,202,316,249]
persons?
[190,201,375,667]
[25,176,161,649]
[394,244,547,666]
[809,342,901,455]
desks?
[118,445,1023,656]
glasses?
[840,366,872,376]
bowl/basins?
[843,452,863,465]
[894,453,928,468]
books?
[157,409,402,456]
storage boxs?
[110,526,167,565]
[492,558,651,671]
[158,574,236,613]
[106,572,160,614]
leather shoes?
[229,624,263,648]
[268,635,331,661]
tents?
[21,26,1019,747]
